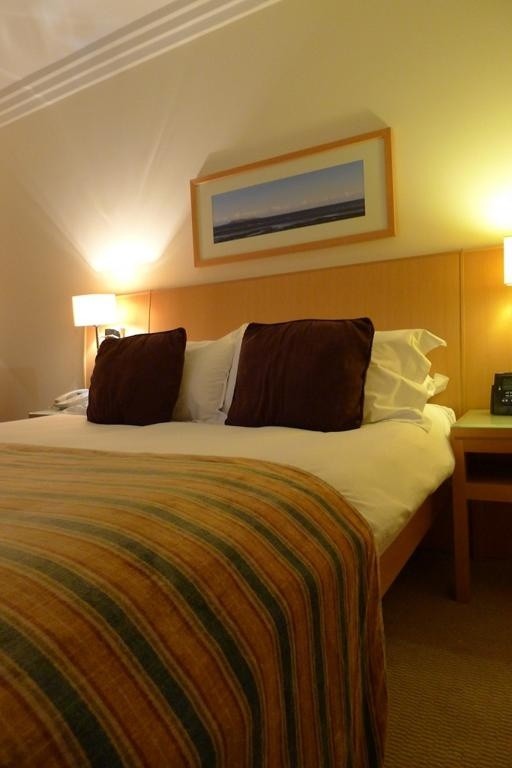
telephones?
[53,388,89,410]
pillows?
[225,317,375,433]
[173,323,248,425]
[87,327,187,426]
[225,323,447,434]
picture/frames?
[190,127,397,269]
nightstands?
[450,410,512,597]
[27,409,61,417]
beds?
[1,243,512,766]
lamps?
[503,236,512,287]
[72,294,121,349]
